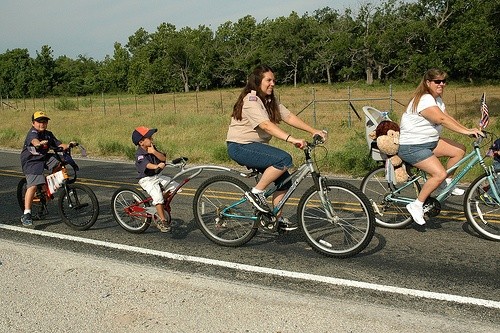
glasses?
[431,78,446,84]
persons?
[20,111,69,227]
[397,69,485,225]
[226,64,327,231]
[132,126,182,232]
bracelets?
[285,134,291,142]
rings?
[322,129,327,134]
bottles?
[430,181,447,197]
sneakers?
[430,179,465,198]
[245,187,271,213]
[479,193,495,207]
[406,202,427,226]
[278,215,301,231]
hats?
[32,110,50,121]
[132,126,158,146]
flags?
[479,93,489,128]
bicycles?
[16,142,99,231]
[359,129,500,242]
[111,134,376,259]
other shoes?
[21,213,33,227]
[157,220,172,233]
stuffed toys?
[370,120,408,184]
